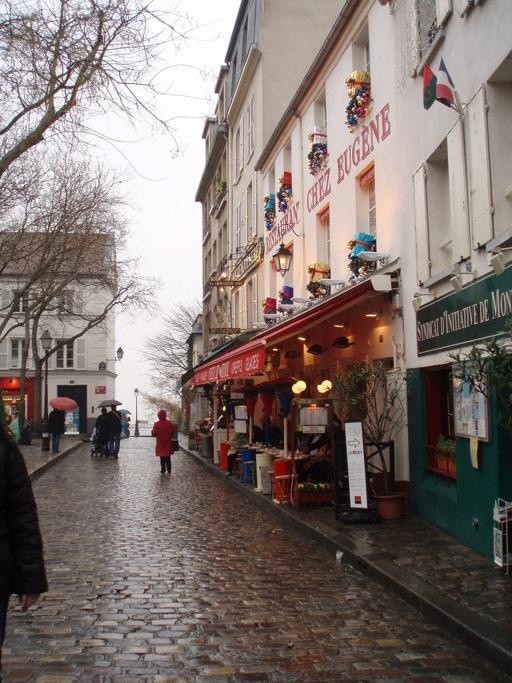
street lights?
[134,387,139,436]
[39,330,53,425]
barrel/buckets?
[219,442,231,471]
[256,453,274,492]
[240,448,257,484]
[271,460,295,503]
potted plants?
[333,361,415,520]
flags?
[422,64,437,111]
[435,57,456,105]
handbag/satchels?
[170,434,179,451]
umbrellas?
[49,397,78,411]
[117,409,132,414]
[97,399,122,408]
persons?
[94,404,129,460]
[150,409,175,473]
[0,389,49,681]
[49,407,66,454]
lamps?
[105,347,124,361]
[411,246,512,312]
[252,251,390,358]
[317,374,335,393]
[271,224,305,277]
[292,375,310,394]
